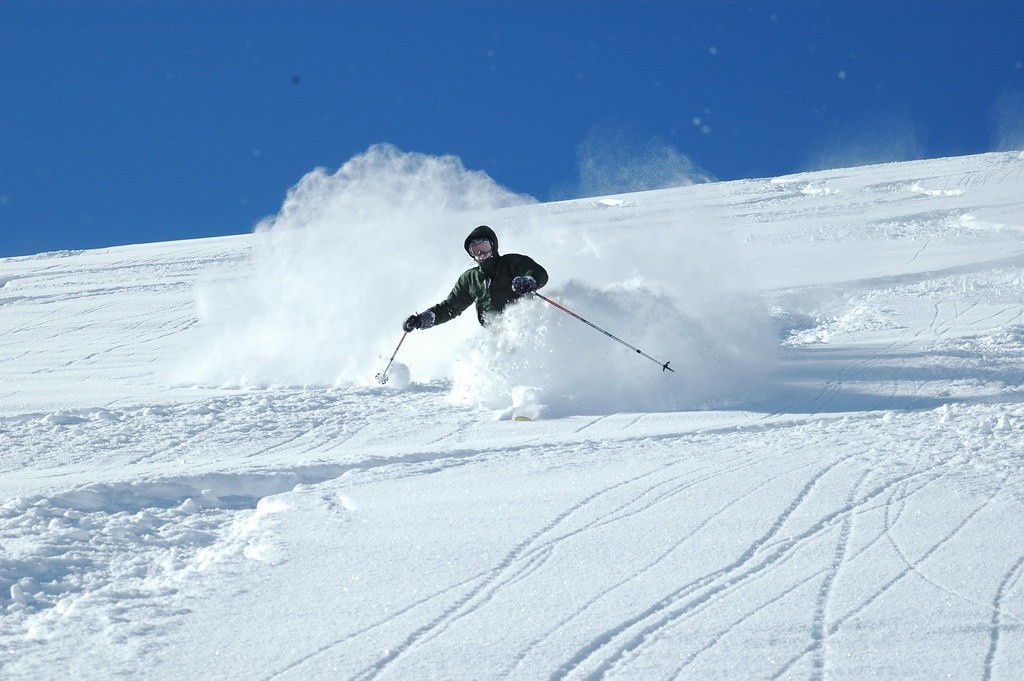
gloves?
[512,276,537,294]
[402,315,422,332]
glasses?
[468,238,494,256]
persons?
[403,226,548,332]
